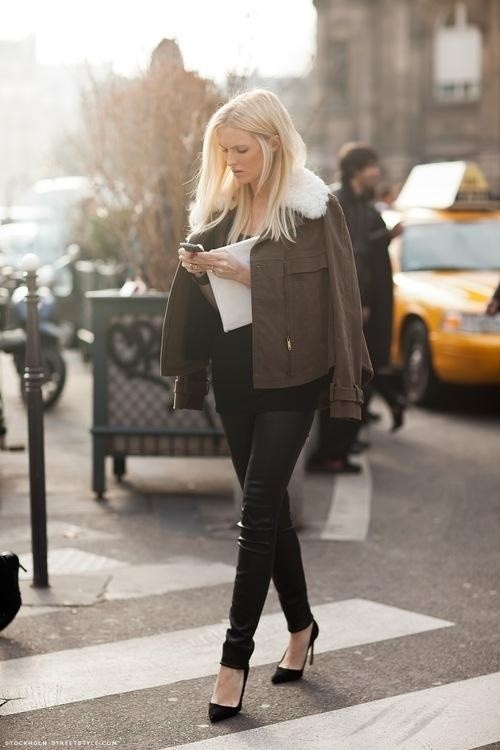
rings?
[209,265,214,272]
[189,263,197,270]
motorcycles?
[1,241,81,413]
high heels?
[271,618,320,689]
[205,659,251,724]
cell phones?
[179,241,203,253]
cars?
[0,176,172,348]
[375,160,500,406]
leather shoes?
[328,400,408,474]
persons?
[487,280,500,318]
[160,89,374,724]
[305,142,406,474]
